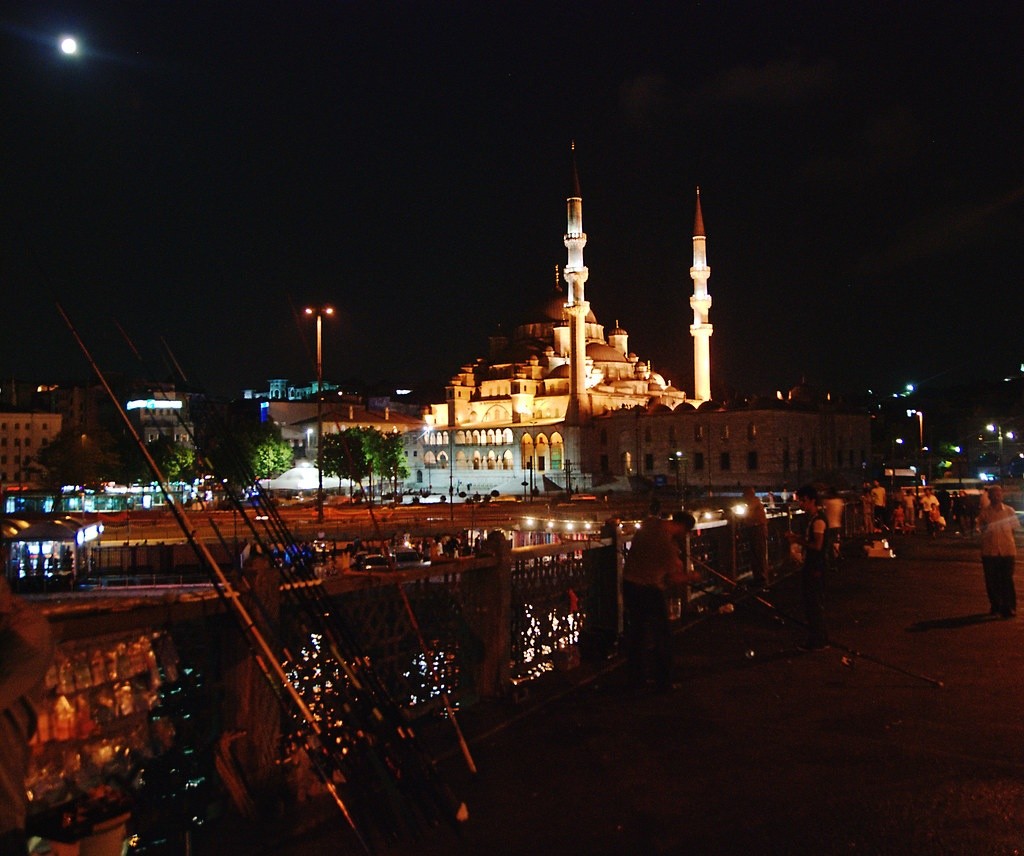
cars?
[356,552,390,573]
[390,546,434,570]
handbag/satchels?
[779,537,807,572]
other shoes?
[1003,608,1017,618]
[795,642,826,653]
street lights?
[305,304,337,523]
[424,426,434,491]
[305,428,312,451]
[986,423,1015,488]
[674,451,683,500]
[36,384,58,442]
[907,409,925,478]
[530,421,539,491]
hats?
[673,512,695,533]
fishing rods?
[48,291,482,855]
[684,580,801,631]
[683,552,945,689]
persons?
[859,480,977,544]
[617,511,700,692]
[784,484,833,654]
[975,484,1024,620]
[0,571,54,856]
[820,487,844,561]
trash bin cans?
[39,802,132,855]
